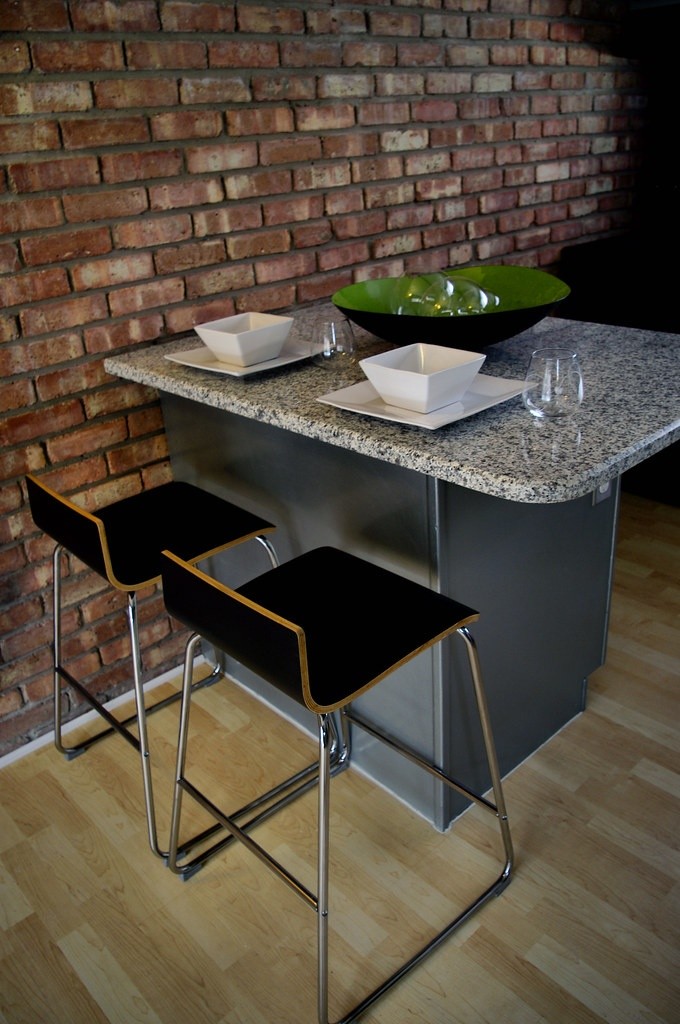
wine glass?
[309,315,357,392]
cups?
[417,276,501,315]
[389,265,456,316]
[522,347,584,417]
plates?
[163,339,335,377]
[315,373,539,431]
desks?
[106,303,680,827]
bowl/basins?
[358,342,487,415]
[193,311,294,368]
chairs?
[164,548,515,1024]
[23,473,339,861]
